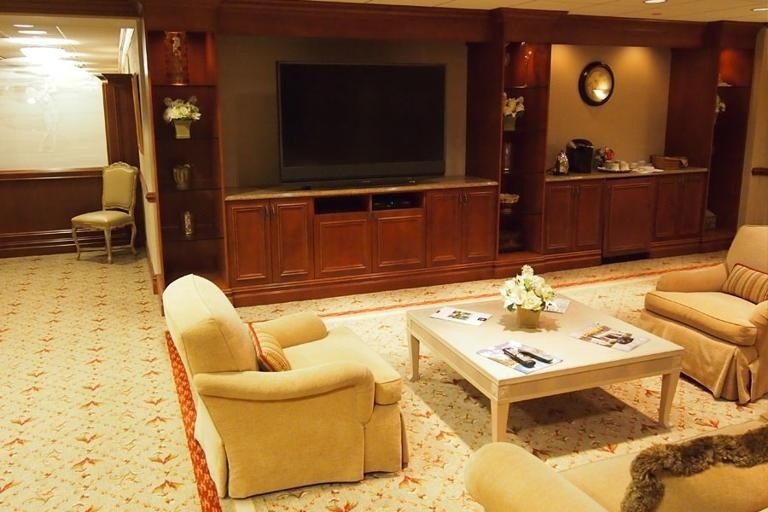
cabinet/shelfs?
[543,174,708,253]
[148,28,227,296]
[227,188,495,286]
[467,41,548,257]
[668,46,755,239]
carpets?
[164,262,767,512]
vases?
[173,118,192,139]
[516,305,540,330]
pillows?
[721,263,768,305]
[248,322,292,372]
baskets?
[650,154,685,170]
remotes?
[519,345,553,364]
[504,348,536,367]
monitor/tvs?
[275,60,446,188]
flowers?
[499,264,556,312]
[162,96,203,122]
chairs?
[463,413,768,512]
[71,161,138,265]
[640,224,768,405]
[160,273,407,501]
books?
[429,306,494,327]
[476,338,564,374]
[541,297,572,314]
[570,320,651,353]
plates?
[597,166,632,173]
